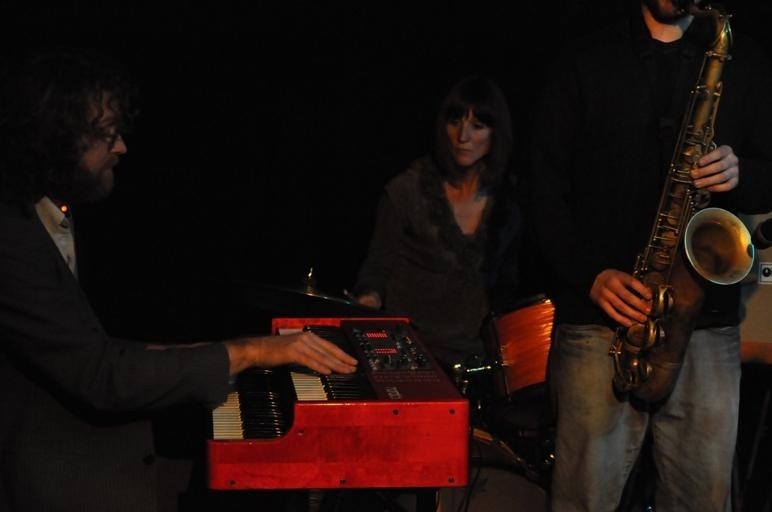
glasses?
[98,123,118,142]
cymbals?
[261,282,354,302]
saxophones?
[608,0,754,413]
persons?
[545,0,750,511]
[1,38,363,512]
[352,75,551,471]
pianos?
[207,317,469,490]
